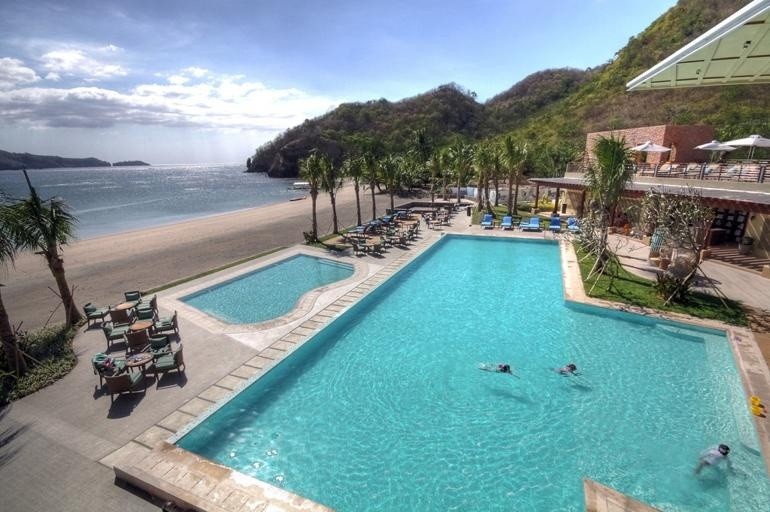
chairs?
[478,211,583,235]
[341,200,459,257]
[628,159,770,181]
[80,289,186,407]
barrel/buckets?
[751,405,761,416]
[750,395,761,406]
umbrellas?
[694,139,736,161]
[724,134,770,159]
[629,140,672,153]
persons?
[552,363,576,377]
[479,363,520,379]
[694,445,730,474]
